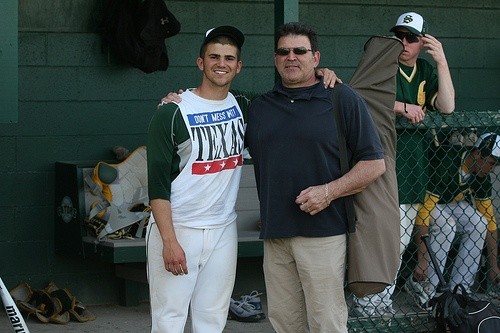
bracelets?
[324,184,330,205]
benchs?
[52,158,265,306]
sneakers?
[237,290,266,319]
[228,299,262,321]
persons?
[405,132,500,312]
[160,23,386,333]
[145,26,342,333]
[355,11,455,316]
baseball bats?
[420,233,446,287]
[0,278,29,333]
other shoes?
[413,296,433,311]
[356,301,394,317]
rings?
[307,207,311,212]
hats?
[389,12,429,36]
[474,132,500,158]
[200,25,244,57]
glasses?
[276,47,315,56]
[395,30,422,43]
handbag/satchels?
[431,285,500,333]
